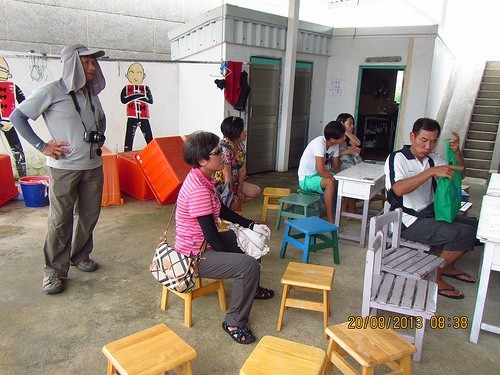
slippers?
[440,270,476,283]
[438,286,465,299]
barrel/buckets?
[18,175,49,207]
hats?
[60,43,105,95]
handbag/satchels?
[149,242,200,292]
[434,139,462,223]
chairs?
[369,185,472,363]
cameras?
[84,130,105,143]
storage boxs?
[134,136,193,206]
[101,146,125,208]
[116,150,154,201]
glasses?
[209,146,224,157]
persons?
[175,132,276,345]
[214,116,261,209]
[325,113,362,220]
[385,118,479,299]
[298,121,347,233]
[9,44,106,291]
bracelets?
[248,221,259,229]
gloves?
[254,224,271,241]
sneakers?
[43,270,63,294]
[70,256,97,272]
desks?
[469,173,500,345]
[334,160,387,249]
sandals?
[221,319,255,343]
[253,285,274,298]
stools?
[297,189,326,215]
[239,336,328,375]
[276,262,335,339]
[161,276,228,328]
[103,323,198,375]
[325,318,417,375]
[262,186,291,222]
[276,194,321,232]
[279,217,341,265]
